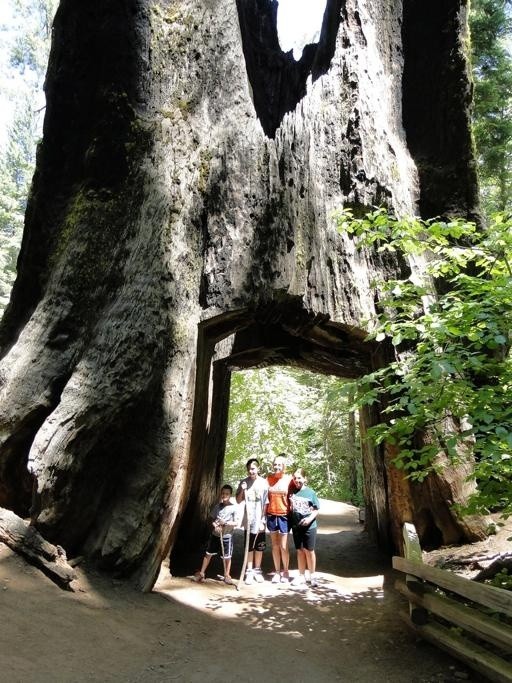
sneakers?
[290,576,306,586]
[225,575,233,585]
[272,573,281,582]
[191,573,205,583]
[281,574,290,583]
[309,576,317,587]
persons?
[288,467,320,587]
[236,459,269,585]
[265,454,293,583]
[191,484,238,584]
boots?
[245,568,254,584]
[254,567,264,582]
